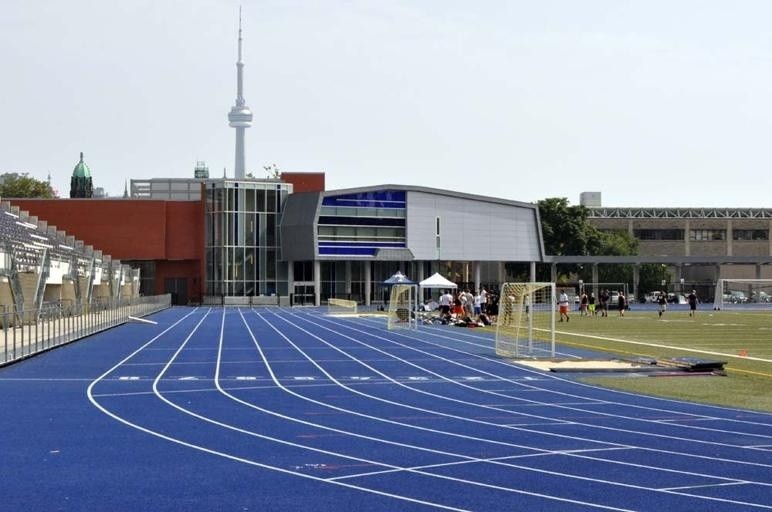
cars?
[576,289,772,310]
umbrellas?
[420,272,457,288]
[382,270,419,286]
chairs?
[0,209,119,272]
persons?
[618,291,626,316]
[439,286,612,327]
[686,289,698,317]
[657,290,669,318]
